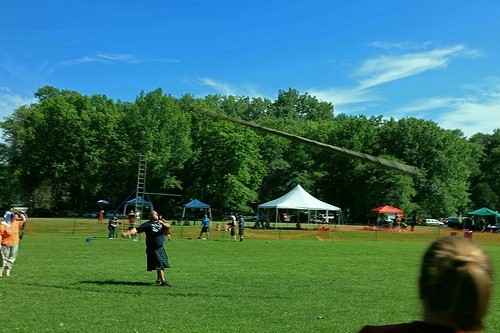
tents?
[123,197,153,215]
[182,199,213,222]
[466,207,500,227]
[371,205,403,227]
[257,184,342,229]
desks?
[193,218,202,225]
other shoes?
[156,280,162,284]
[0,270,2,276]
[161,281,172,287]
[6,269,10,276]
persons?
[198,214,211,239]
[159,216,171,241]
[358,235,494,333]
[238,215,244,242]
[98,210,104,225]
[109,216,120,239]
[121,211,173,288]
[393,215,407,228]
[465,215,485,232]
[128,210,135,226]
[260,211,264,227]
[0,208,28,277]
[228,215,237,241]
